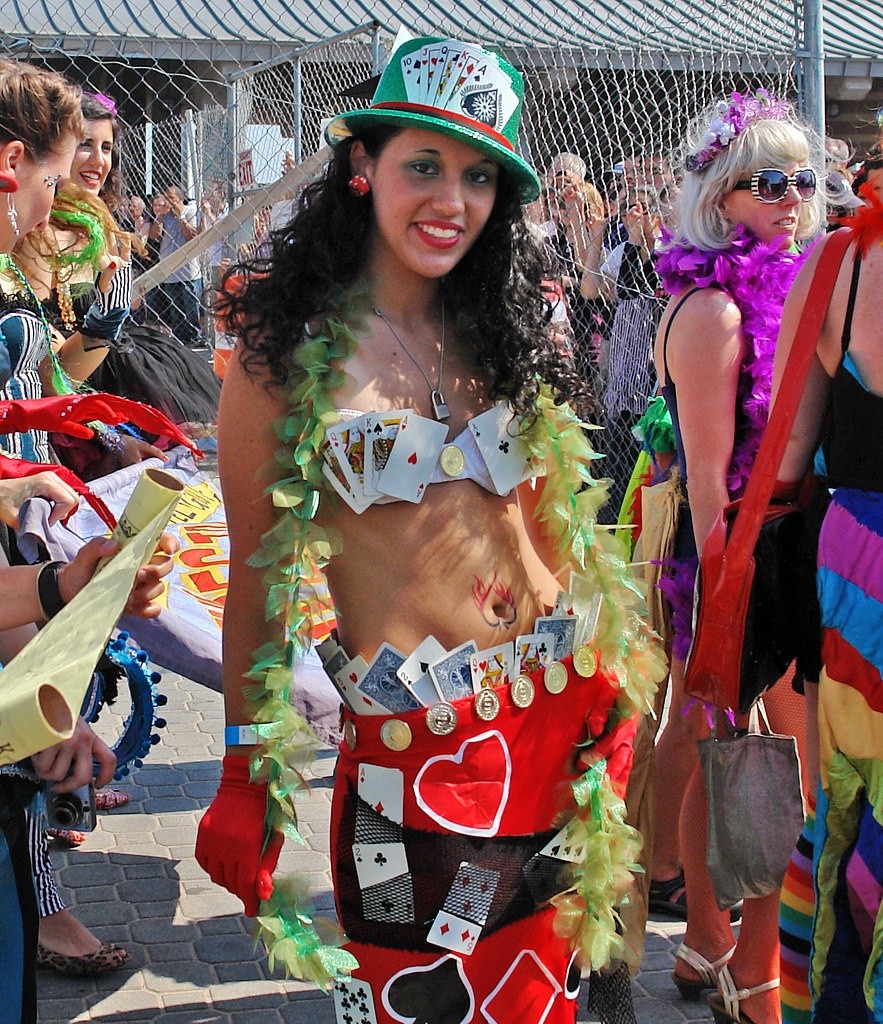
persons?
[0,57,881,1023]
[192,37,640,1024]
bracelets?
[224,720,286,746]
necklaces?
[358,280,447,420]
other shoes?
[49,828,87,847]
[95,788,131,811]
[36,940,133,977]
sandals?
[648,868,745,921]
[672,938,781,1024]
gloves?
[53,397,130,439]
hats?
[324,37,542,205]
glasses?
[728,169,822,204]
[619,202,651,217]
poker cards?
[314,570,602,718]
[356,761,405,847]
[316,408,450,514]
[330,975,376,1024]
[468,402,541,495]
[426,861,500,956]
[353,841,416,924]
[399,38,520,134]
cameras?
[44,769,97,832]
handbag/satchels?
[695,695,806,911]
[683,476,835,716]
